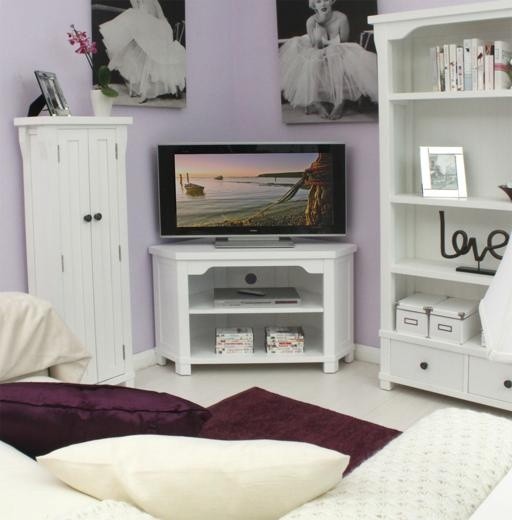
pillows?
[0,383,212,461]
[34,432,351,520]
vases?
[90,89,115,115]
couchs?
[0,290,512,520]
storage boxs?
[395,292,450,338]
[215,327,254,355]
[430,295,481,345]
[264,326,305,355]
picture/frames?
[26,70,72,116]
[418,146,468,198]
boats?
[184,183,204,191]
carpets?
[197,386,402,477]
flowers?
[65,23,120,98]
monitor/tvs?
[157,144,347,247]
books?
[430,38,512,92]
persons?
[277,0,378,119]
[98,0,186,104]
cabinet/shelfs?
[14,116,136,388]
[367,4,511,414]
[147,238,357,376]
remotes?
[237,289,265,297]
